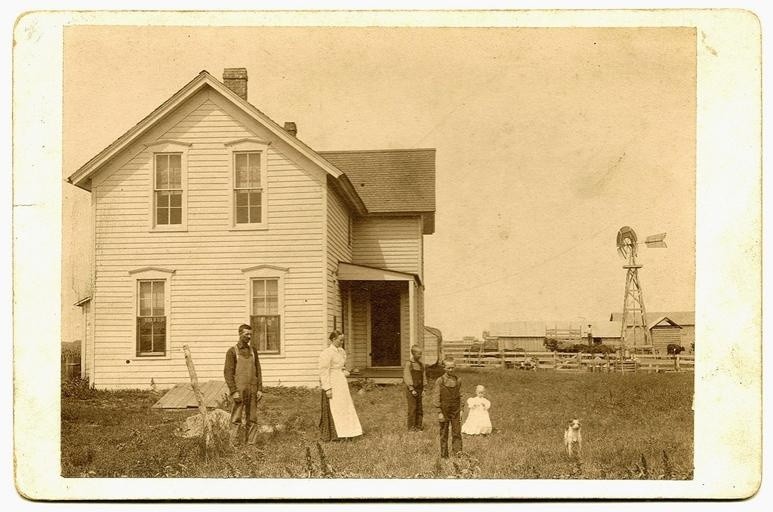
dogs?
[564,417,583,459]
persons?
[462,385,493,436]
[432,358,465,463]
[403,344,428,434]
[222,323,264,446]
[317,329,364,443]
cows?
[666,343,686,356]
